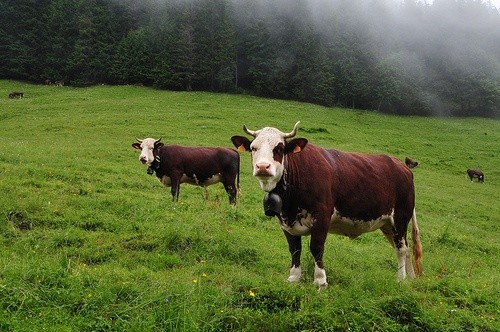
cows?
[132,137,242,208]
[54,81,65,87]
[404,157,419,169]
[8,92,24,100]
[231,121,423,289]
[44,80,52,85]
[466,168,485,183]
